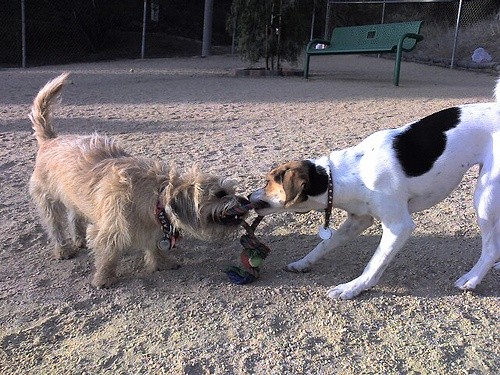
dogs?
[25,70,251,291]
[247,77,500,302]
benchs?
[303,20,425,86]
